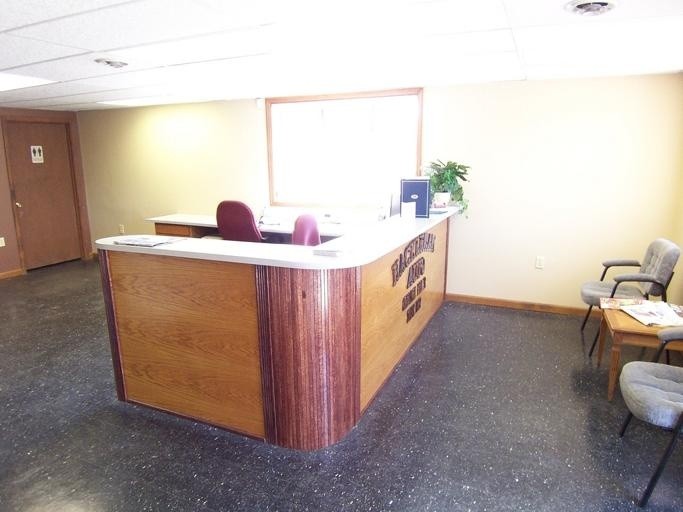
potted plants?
[418,159,471,208]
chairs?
[215,199,272,242]
[290,213,321,247]
[578,236,682,511]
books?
[599,297,683,328]
[112,235,184,248]
